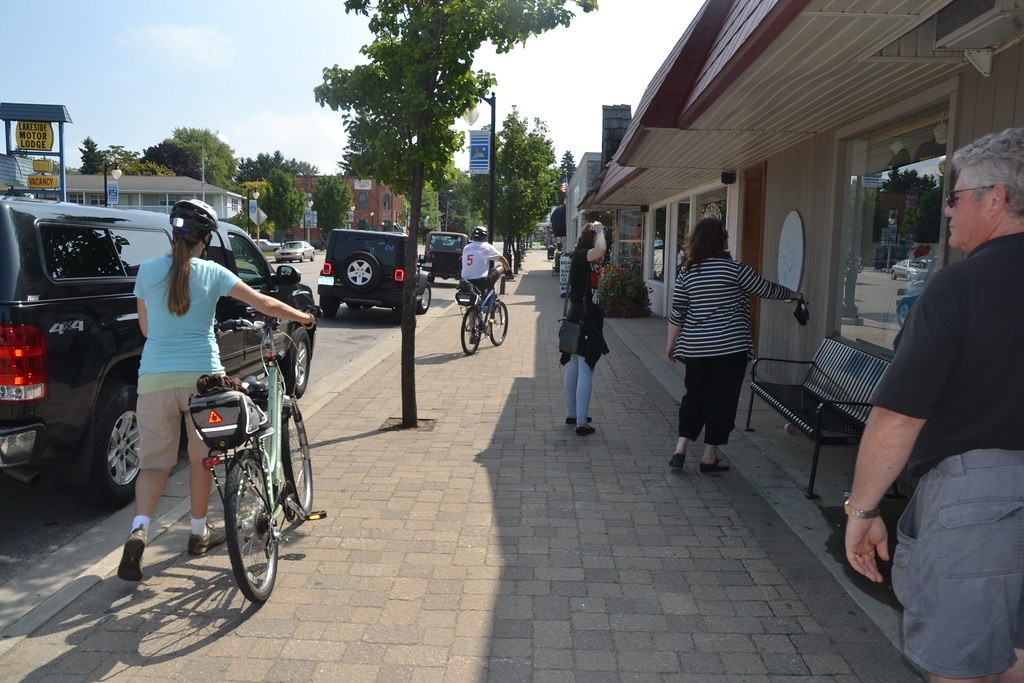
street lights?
[347,205,355,229]
[436,190,453,232]
[889,214,900,260]
[104,162,122,207]
[247,188,260,236]
[304,197,314,241]
[370,210,374,231]
[463,92,494,271]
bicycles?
[201,304,327,604]
[459,289,508,356]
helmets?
[169,199,219,234]
[472,225,489,238]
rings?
[856,555,861,558]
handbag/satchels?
[187,388,262,447]
[558,320,581,353]
[793,294,809,325]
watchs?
[844,498,881,518]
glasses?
[944,185,1008,208]
[723,230,729,240]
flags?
[560,176,568,192]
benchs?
[744,335,910,499]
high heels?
[669,453,686,469]
[699,456,729,474]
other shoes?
[565,416,592,425]
[470,335,478,344]
[576,423,595,436]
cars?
[890,259,929,281]
[876,241,898,269]
[252,239,285,252]
[275,241,315,263]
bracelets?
[596,229,604,233]
[301,314,315,329]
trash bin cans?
[548,246,554,259]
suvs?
[317,229,432,318]
[422,231,469,282]
[896,255,939,328]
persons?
[844,126,1024,683]
[560,220,610,435]
[666,218,806,472]
[460,226,510,344]
[118,198,316,581]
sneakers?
[188,522,225,555]
[117,524,147,580]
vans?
[0,195,324,511]
